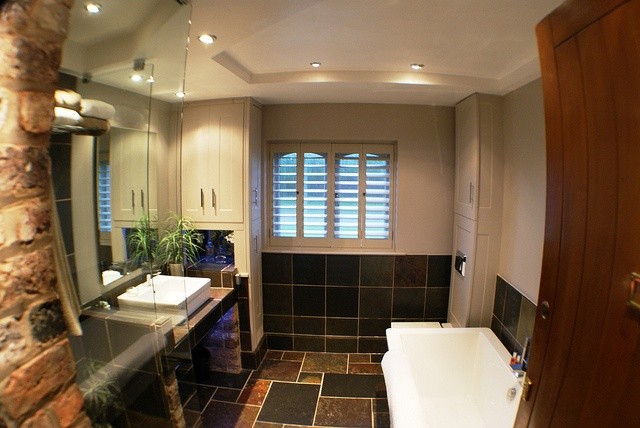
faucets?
[514,371,525,379]
[146,272,161,286]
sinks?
[116,275,212,320]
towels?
[53,90,80,110]
[43,164,85,336]
[80,98,114,119]
[49,106,79,127]
[81,116,110,130]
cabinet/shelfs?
[182,96,250,230]
[448,214,501,329]
[110,128,163,229]
[249,219,265,350]
[445,93,503,219]
[246,96,265,221]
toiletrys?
[510,351,522,364]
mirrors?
[50,72,170,317]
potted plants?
[156,211,200,278]
[76,360,125,428]
[121,220,160,271]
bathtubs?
[379,327,523,428]
